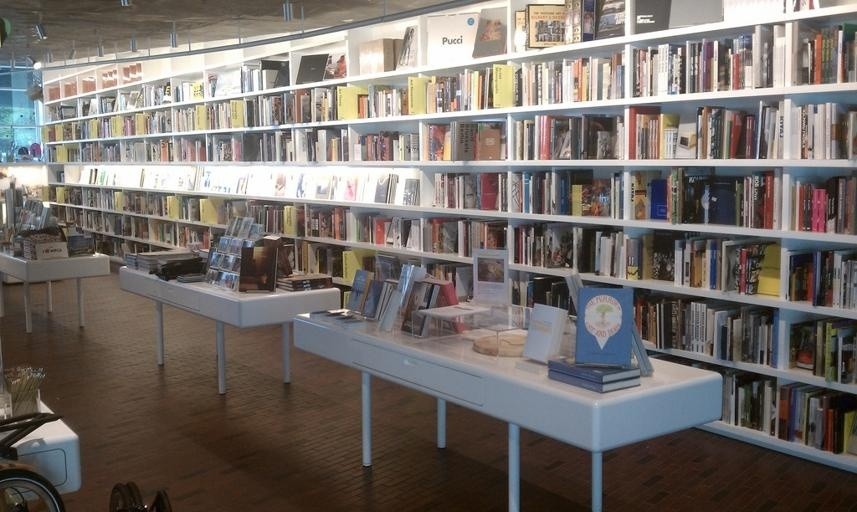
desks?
[118,262,340,396]
[289,301,722,511]
[0,390,81,505]
[1,248,111,334]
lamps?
[35,25,50,41]
[281,2,293,23]
[130,38,138,53]
[96,44,106,58]
[169,33,179,49]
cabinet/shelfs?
[40,4,857,474]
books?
[49,167,198,256]
[793,101,857,160]
[722,369,778,437]
[49,61,142,101]
[792,170,857,235]
[512,50,625,105]
[348,7,507,162]
[634,290,779,374]
[126,236,346,294]
[512,167,624,220]
[625,231,780,298]
[778,382,857,456]
[629,167,782,230]
[175,51,349,164]
[523,286,654,393]
[801,23,857,84]
[785,247,857,311]
[430,167,509,255]
[512,224,624,278]
[348,262,469,338]
[46,80,172,163]
[628,99,784,160]
[198,165,423,253]
[789,317,857,385]
[515,114,624,160]
[632,24,787,97]
